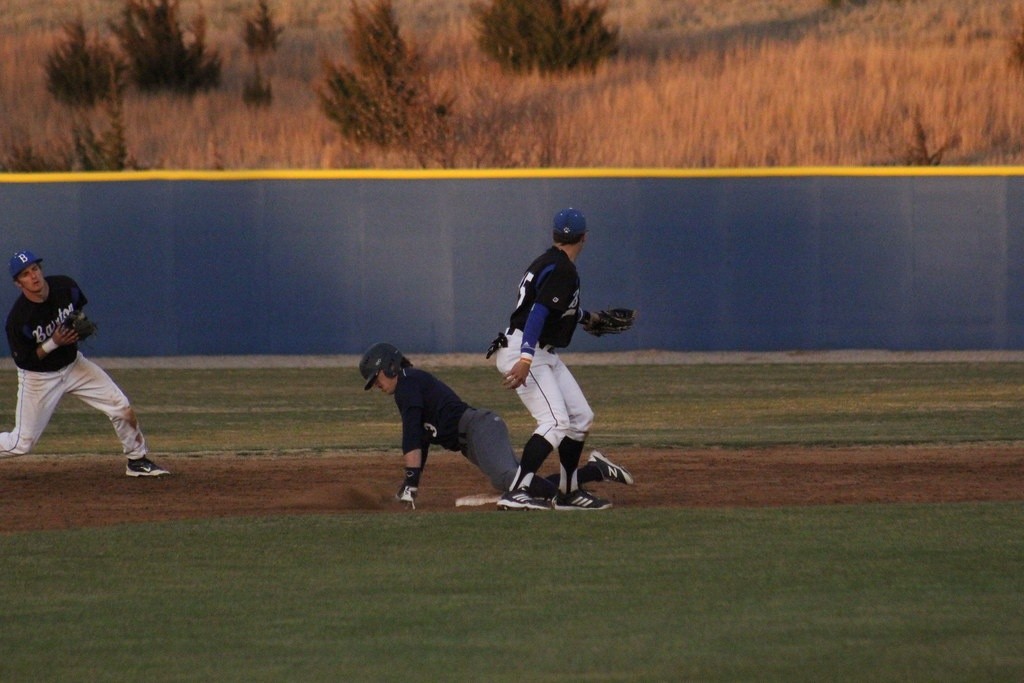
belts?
[509,327,554,353]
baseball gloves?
[60,309,97,342]
[582,308,638,338]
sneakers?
[588,448,634,485]
[555,488,613,511]
[496,488,551,511]
[126,455,171,478]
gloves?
[396,468,420,510]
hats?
[9,251,43,276]
[553,208,588,235]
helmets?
[359,343,403,391]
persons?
[485,207,638,511]
[1,250,172,478]
[358,341,635,511]
